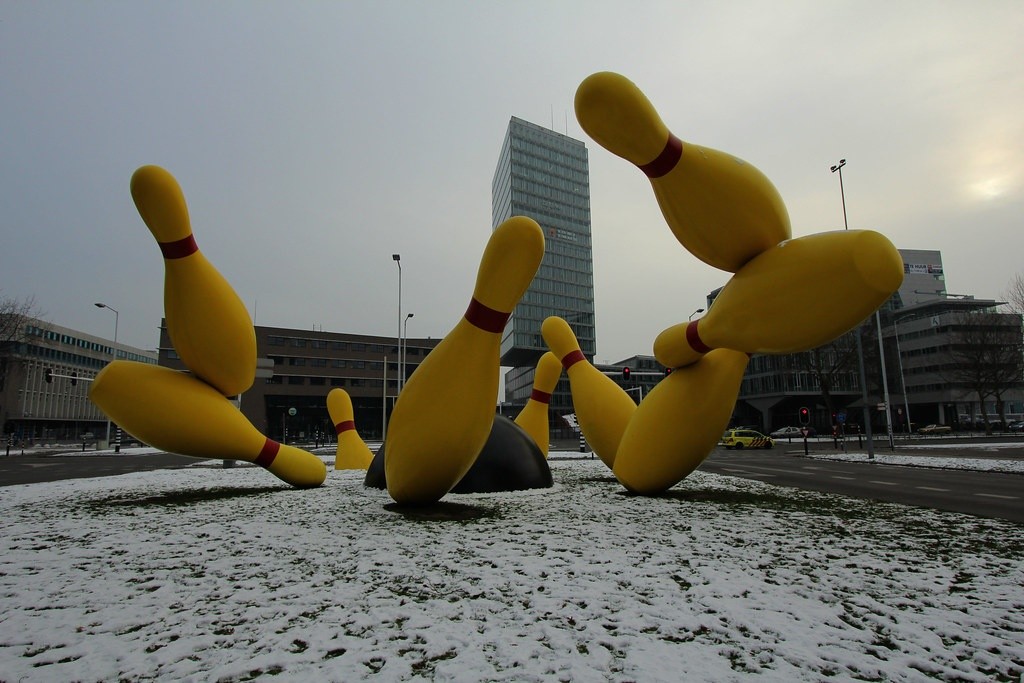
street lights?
[392,253,402,395]
[830,157,848,231]
[894,313,917,432]
[95,302,119,447]
[402,313,415,387]
[688,308,704,321]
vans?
[722,430,775,449]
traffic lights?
[830,412,837,428]
[800,407,810,425]
[664,367,672,377]
[573,413,578,425]
[623,367,631,381]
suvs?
[80,432,94,440]
[770,426,803,439]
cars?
[1006,422,1024,432]
[917,423,952,435]
